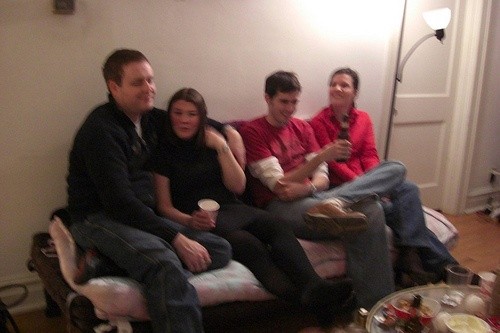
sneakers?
[303,203,368,229]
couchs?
[48,207,460,321]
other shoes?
[72,248,108,285]
[301,276,352,313]
[453,267,480,285]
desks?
[366,283,500,333]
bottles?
[335,113,351,162]
[400,295,426,333]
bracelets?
[217,143,230,155]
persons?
[239,71,407,311]
[307,68,478,290]
[65,49,247,333]
[153,88,360,325]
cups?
[197,198,220,224]
[444,313,493,332]
[444,264,473,306]
[478,271,495,301]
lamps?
[383,0,450,161]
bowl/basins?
[390,292,441,326]
